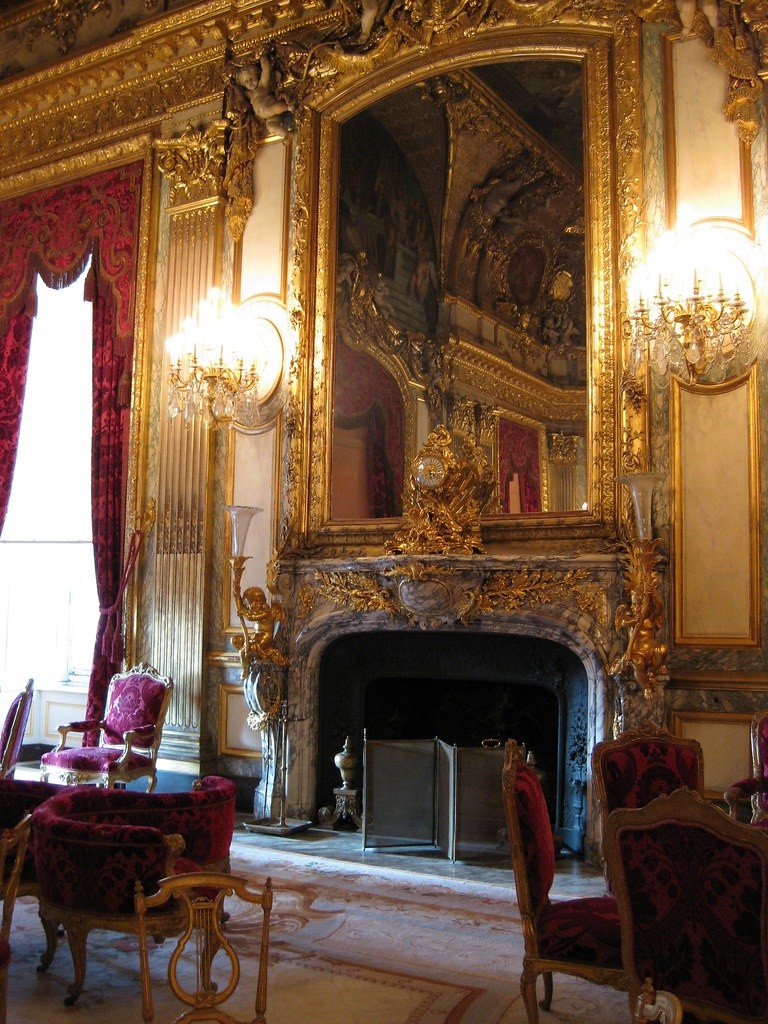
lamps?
[166,287,287,439]
[622,218,768,387]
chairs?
[0,814,32,950]
[724,708,768,827]
[32,800,229,1009]
[593,729,705,898]
[41,661,171,798]
[59,774,236,943]
[499,740,626,1024]
[134,869,274,1024]
[0,678,35,781]
[604,787,768,1024]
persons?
[233,54,296,138]
[357,0,379,47]
[615,585,668,698]
[373,279,395,319]
[673,0,721,38]
[230,586,275,680]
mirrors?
[309,32,611,537]
